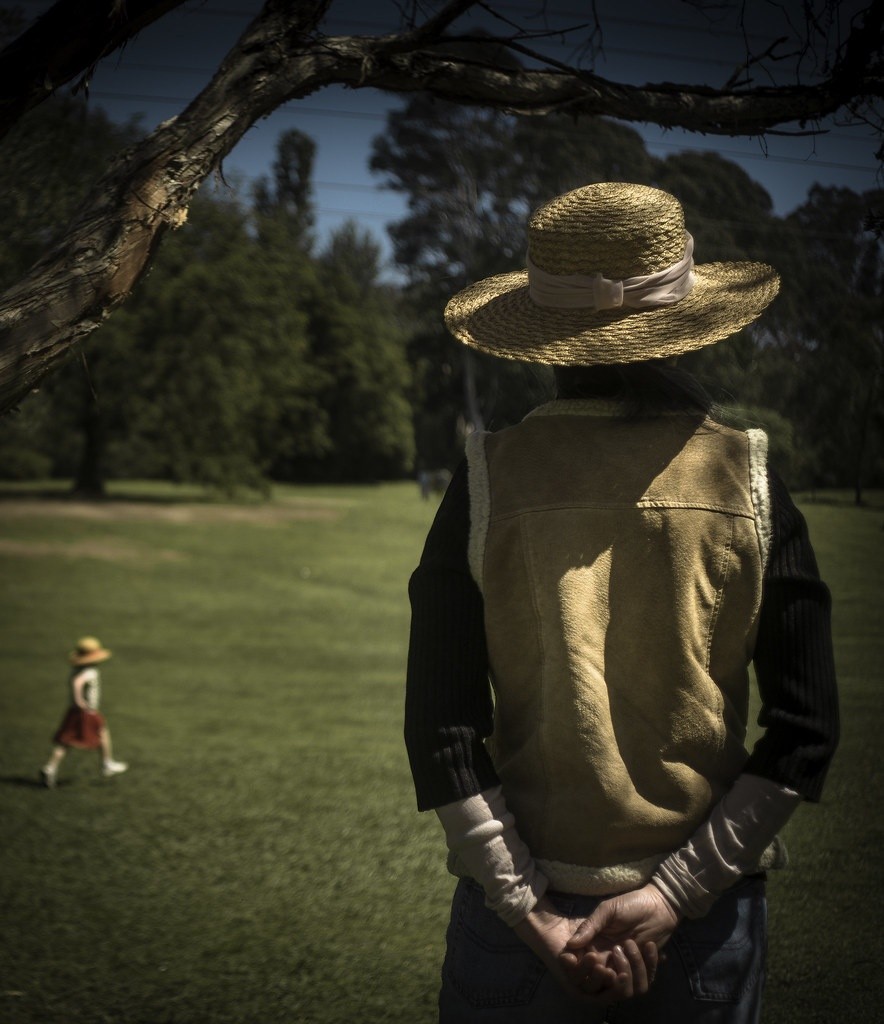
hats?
[446,182,780,365]
[68,638,110,664]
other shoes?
[42,767,55,786]
[102,762,130,776]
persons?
[400,181,844,1023]
[38,635,131,792]
